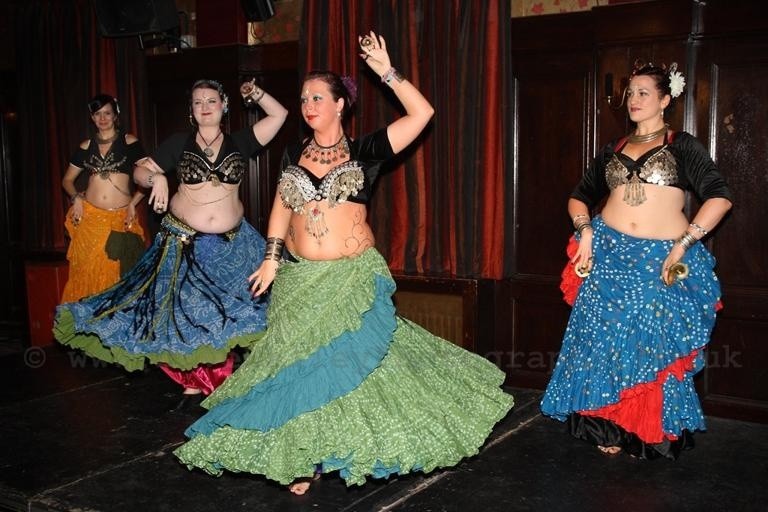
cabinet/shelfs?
[504,0,767,431]
[143,38,304,239]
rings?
[369,46,374,51]
[588,256,592,263]
[258,281,264,288]
[364,54,369,62]
[665,266,669,269]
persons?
[170,29,516,497]
[49,76,289,397]
[540,65,735,463]
[58,94,150,306]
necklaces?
[198,130,222,157]
[628,124,670,146]
[96,130,119,145]
[302,133,350,165]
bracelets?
[677,223,707,252]
[572,213,592,233]
[249,85,265,104]
[264,236,286,265]
[70,192,83,203]
[381,67,404,89]
[148,172,155,185]
